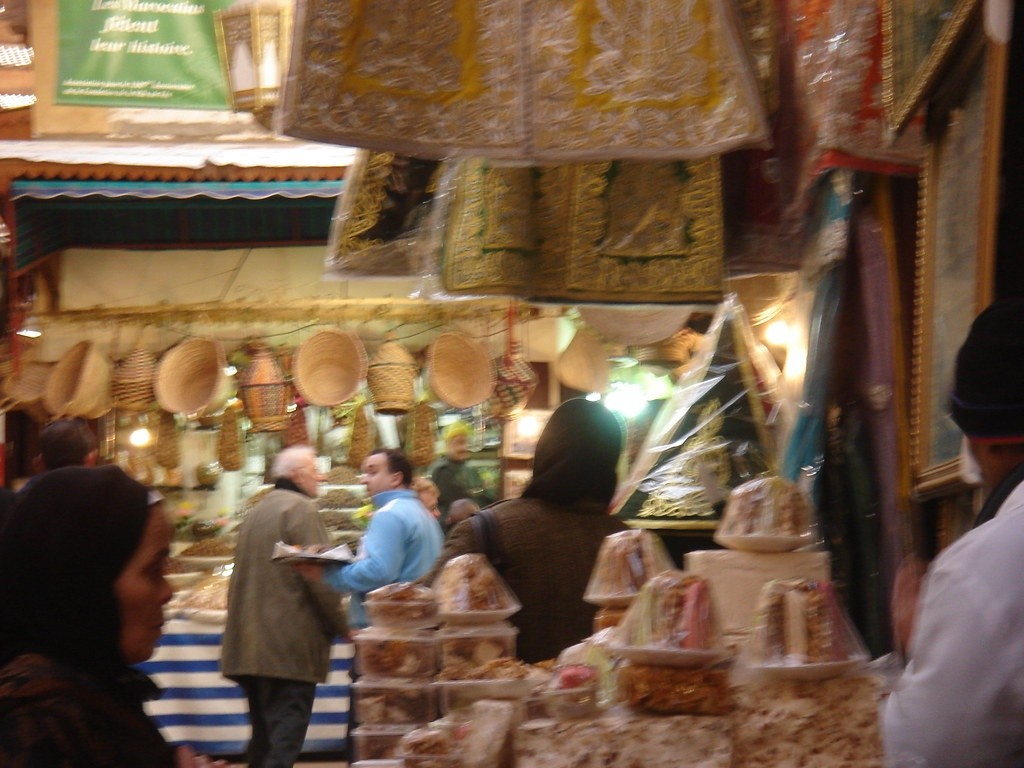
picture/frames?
[908,31,1005,506]
[502,469,533,500]
[503,407,556,461]
[880,0,982,137]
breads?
[354,475,886,768]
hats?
[951,296,1024,442]
[446,424,468,438]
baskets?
[0,332,536,421]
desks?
[128,610,357,761]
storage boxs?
[345,726,419,761]
[359,597,440,632]
[436,622,521,675]
[431,681,523,719]
[346,676,435,725]
[350,625,440,680]
[683,548,832,635]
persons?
[805,402,871,651]
[0,404,230,768]
[412,479,442,524]
[879,301,1024,768]
[215,444,357,768]
[437,396,636,667]
[299,446,444,766]
